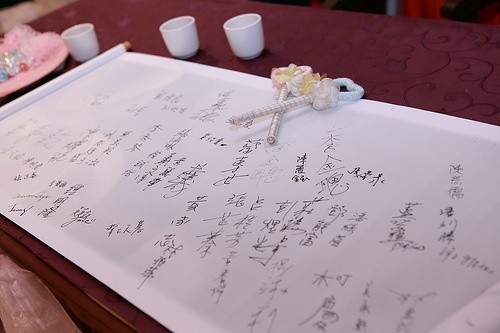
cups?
[61,23,100,62]
[223,13,265,59]
[159,15,199,59]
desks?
[0,0,500,333]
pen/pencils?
[225,63,364,144]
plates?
[1,33,68,98]
